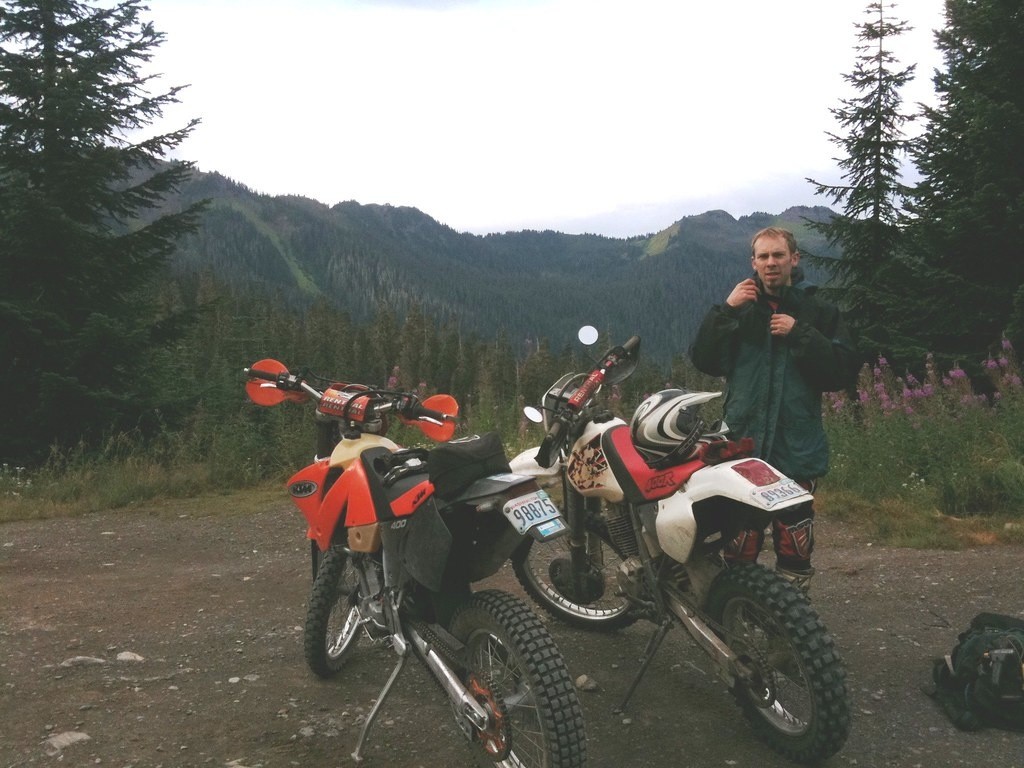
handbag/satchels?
[428,432,512,501]
[933,625,1024,733]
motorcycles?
[243,357,587,767]
[504,324,852,764]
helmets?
[629,388,731,469]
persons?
[688,226,859,640]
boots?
[775,562,815,603]
